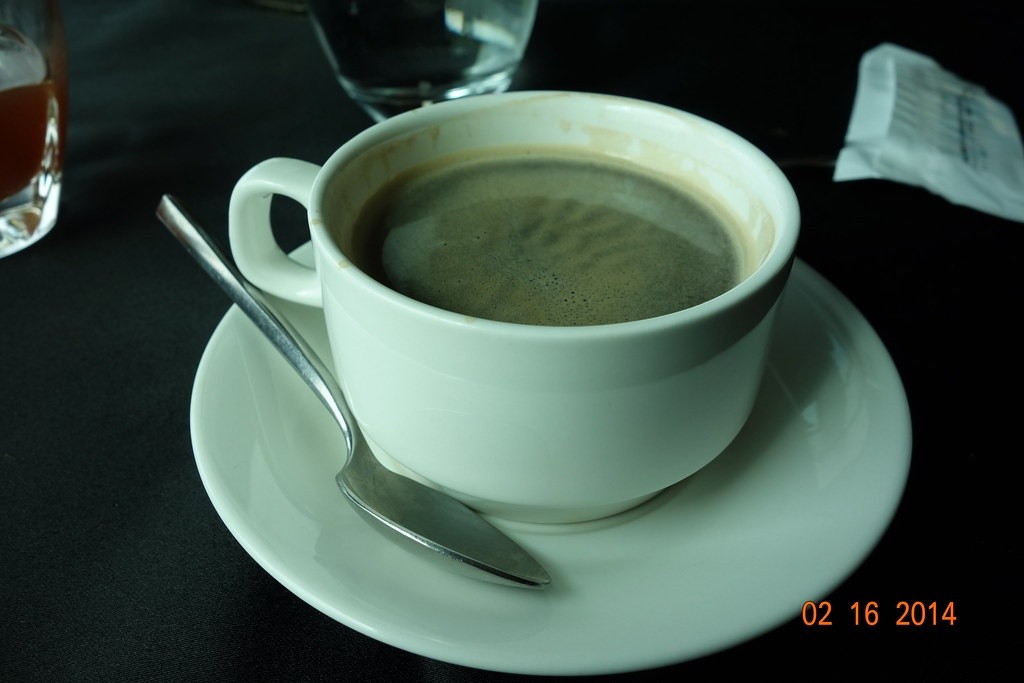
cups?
[296,0,539,128]
[0,0,66,258]
[227,91,801,527]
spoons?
[154,193,553,592]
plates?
[190,238,914,676]
